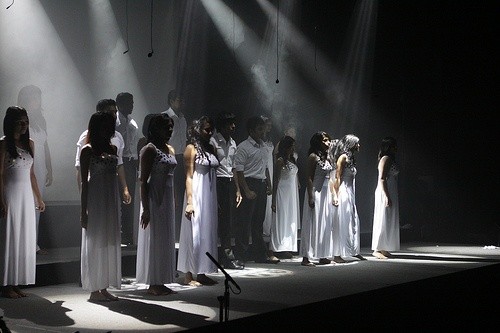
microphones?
[276,80,279,83]
[206,251,241,291]
[148,52,153,57]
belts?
[215,177,234,182]
[246,179,266,184]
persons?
[273,119,301,233]
[0,105,46,298]
[208,111,246,271]
[113,91,139,250]
[370,135,402,260]
[257,114,276,237]
[137,112,161,169]
[233,115,279,265]
[134,111,179,296]
[297,130,369,267]
[78,111,123,302]
[74,98,132,289]
[164,88,189,239]
[268,134,304,261]
[17,85,54,256]
[176,111,221,287]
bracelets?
[38,200,44,203]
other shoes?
[255,253,280,264]
[224,259,244,270]
[231,257,245,266]
